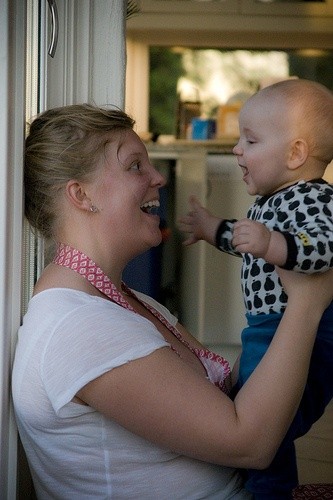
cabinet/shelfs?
[138,140,333,345]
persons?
[10,103,333,500]
[176,79,333,500]
